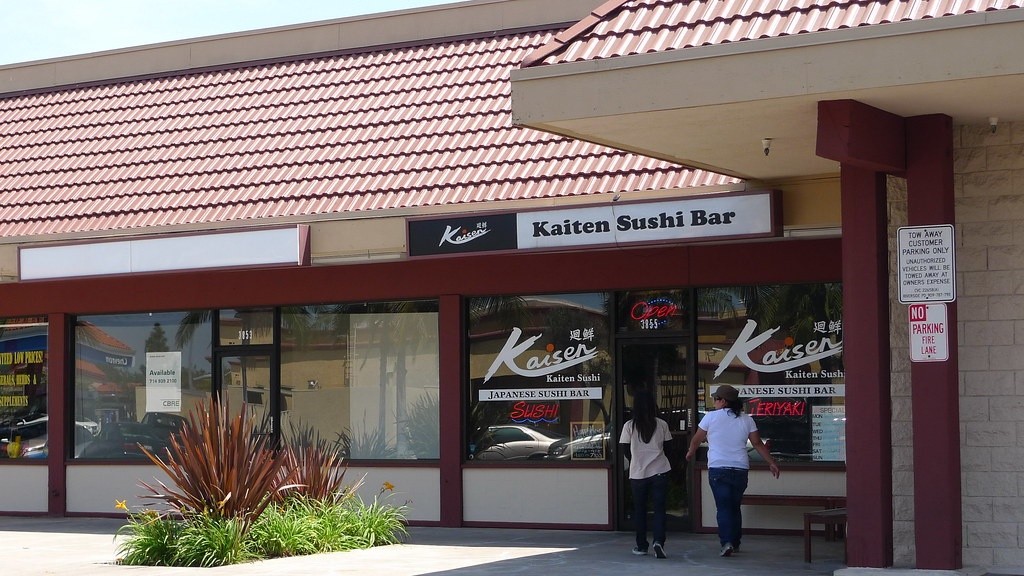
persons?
[618,391,673,558]
[685,384,781,557]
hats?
[711,385,739,401]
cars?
[542,409,774,465]
[0,408,194,461]
[467,424,572,462]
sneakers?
[632,545,646,555]
[652,542,667,559]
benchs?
[803,507,847,564]
[740,494,849,541]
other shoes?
[734,546,740,552]
[720,542,734,557]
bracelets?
[688,448,690,450]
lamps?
[989,116,998,136]
[762,139,772,158]
